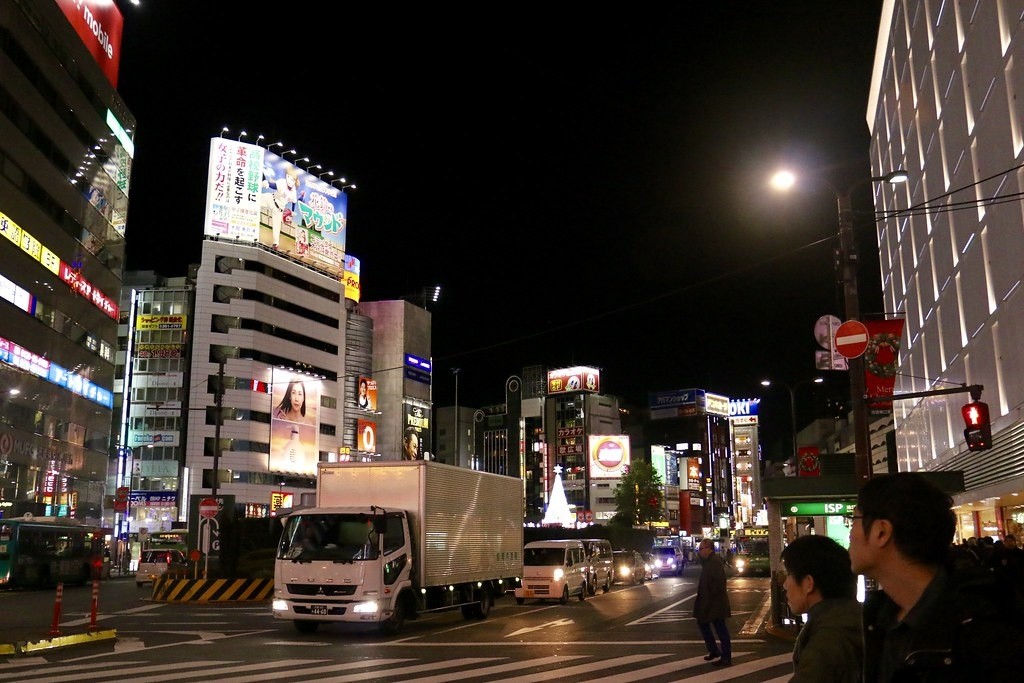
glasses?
[843,515,863,528]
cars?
[135,548,186,588]
[613,550,659,586]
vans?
[514,539,588,605]
[579,539,614,596]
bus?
[732,527,770,577]
[0,512,105,590]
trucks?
[270,459,526,638]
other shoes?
[712,660,731,666]
[704,654,719,660]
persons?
[274,377,312,424]
[682,538,733,667]
[848,471,1024,683]
[358,380,372,410]
[779,535,868,683]
[259,166,306,255]
[404,426,420,462]
[104,548,131,578]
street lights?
[123,443,154,567]
[759,375,825,476]
[451,367,462,466]
[769,166,909,496]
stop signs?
[835,319,871,360]
[198,498,220,519]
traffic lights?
[961,401,993,453]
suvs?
[650,545,685,577]
